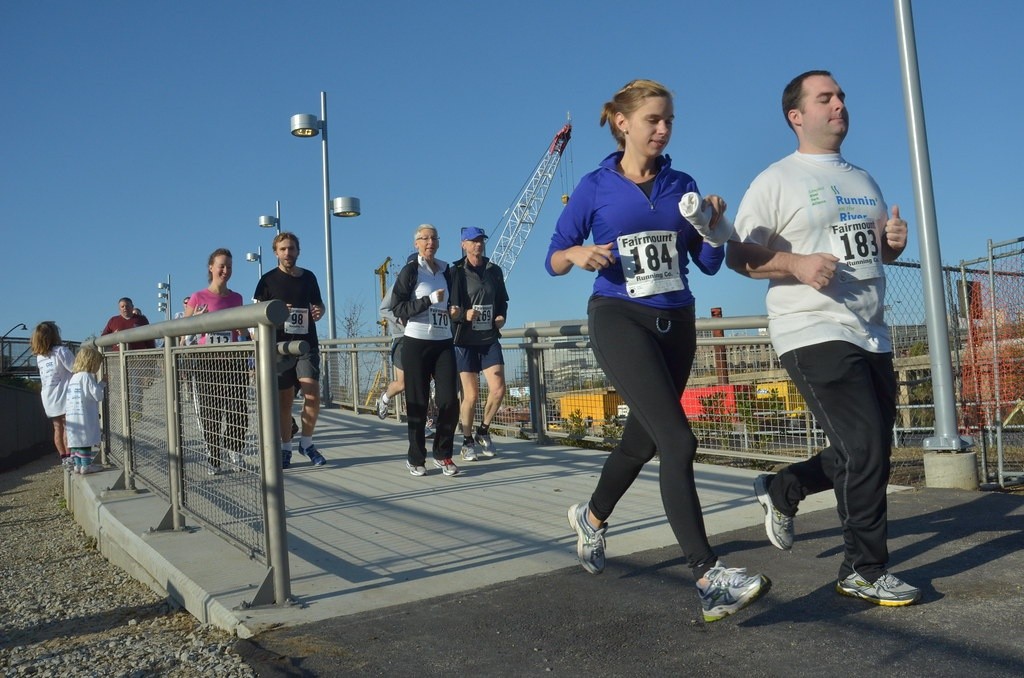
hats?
[460,227,488,241]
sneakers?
[406,460,428,476]
[229,451,245,471]
[754,474,793,551]
[378,392,389,419]
[566,500,608,575]
[837,569,920,606]
[475,430,497,457]
[298,441,326,466]
[425,427,435,438]
[207,464,222,475]
[433,458,459,475]
[281,449,292,468]
[696,560,771,622]
[460,443,479,461]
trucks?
[751,380,810,417]
[682,384,755,428]
[551,391,619,427]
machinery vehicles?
[430,120,574,418]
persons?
[251,296,299,439]
[254,232,326,470]
[391,223,460,477]
[545,81,772,622]
[450,226,509,462]
[66,349,108,474]
[175,297,191,346]
[32,321,75,464]
[379,253,435,439]
[725,70,921,606]
[101,297,156,421]
[183,248,248,475]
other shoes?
[128,411,144,422]
[62,458,73,468]
[80,464,104,474]
[74,465,80,473]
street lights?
[246,246,261,281]
[2,323,28,371]
[290,91,362,404]
[158,275,172,320]
[259,201,281,236]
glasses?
[417,236,439,241]
